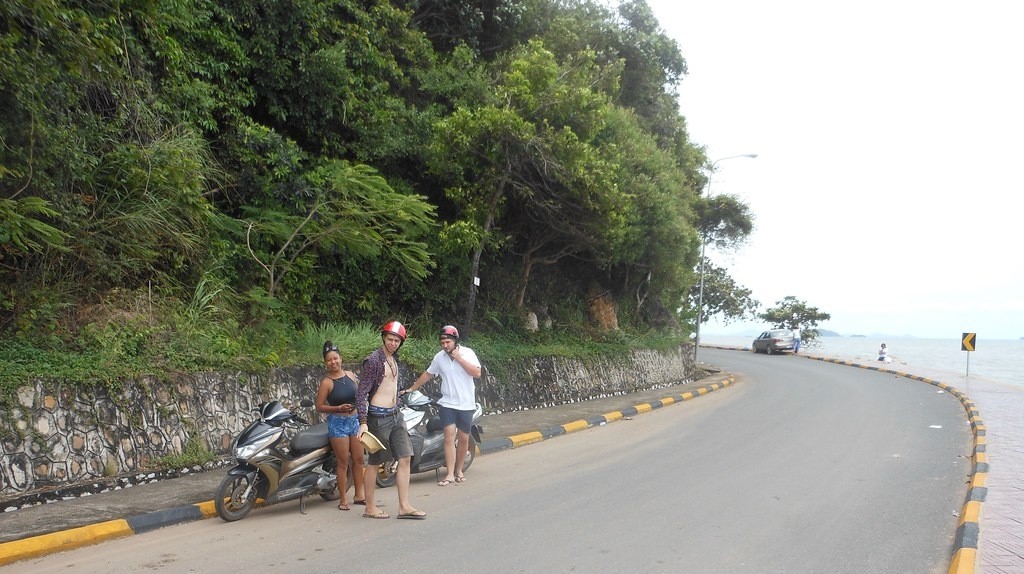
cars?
[752,328,796,356]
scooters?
[216,397,354,523]
[375,390,491,487]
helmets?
[382,321,406,342]
[439,325,459,339]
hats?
[359,430,387,455]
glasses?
[323,345,339,353]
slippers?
[397,510,428,519]
[363,511,389,519]
[354,499,366,505]
[338,504,350,510]
[455,475,465,482]
[439,480,454,486]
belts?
[368,408,399,418]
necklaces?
[386,358,397,377]
[336,376,346,385]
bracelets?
[338,406,342,413]
[409,388,413,392]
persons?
[354,321,427,520]
[398,325,482,486]
[316,341,367,511]
[792,326,800,355]
[878,343,887,361]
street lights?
[694,154,759,363]
